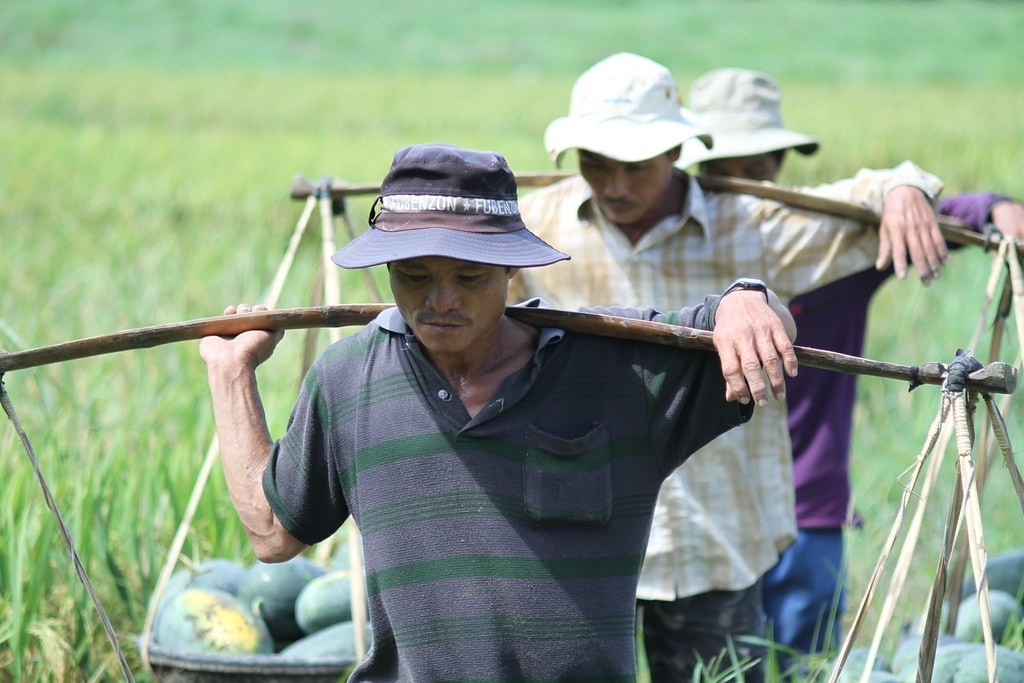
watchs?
[713,278,769,330]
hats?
[545,51,714,167]
[331,142,571,267]
[673,66,818,169]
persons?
[680,68,1024,657]
[198,144,798,683]
[504,53,948,683]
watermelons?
[148,553,374,662]
[827,552,1024,683]
